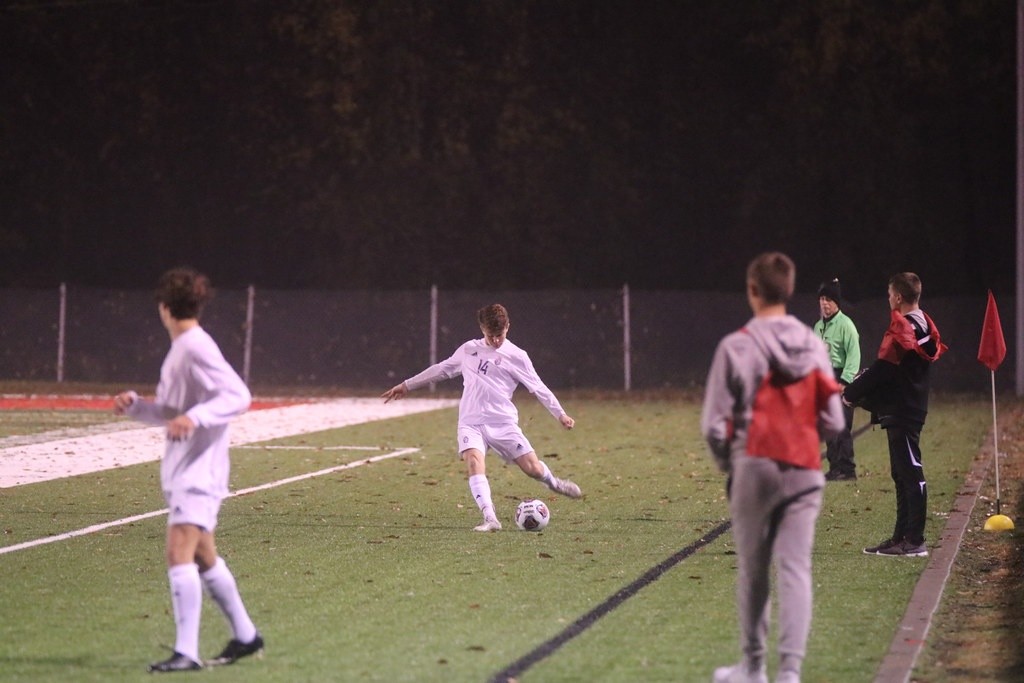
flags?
[977,291,1006,370]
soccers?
[514,498,549,533]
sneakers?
[876,539,928,557]
[474,517,502,532]
[863,536,905,554]
[546,477,581,498]
[147,640,205,674]
[205,635,264,666]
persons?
[110,266,265,674]
[381,303,583,532]
[813,275,861,479]
[842,272,948,557]
[700,252,845,683]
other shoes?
[826,471,857,481]
[713,666,735,683]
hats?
[818,278,842,309]
